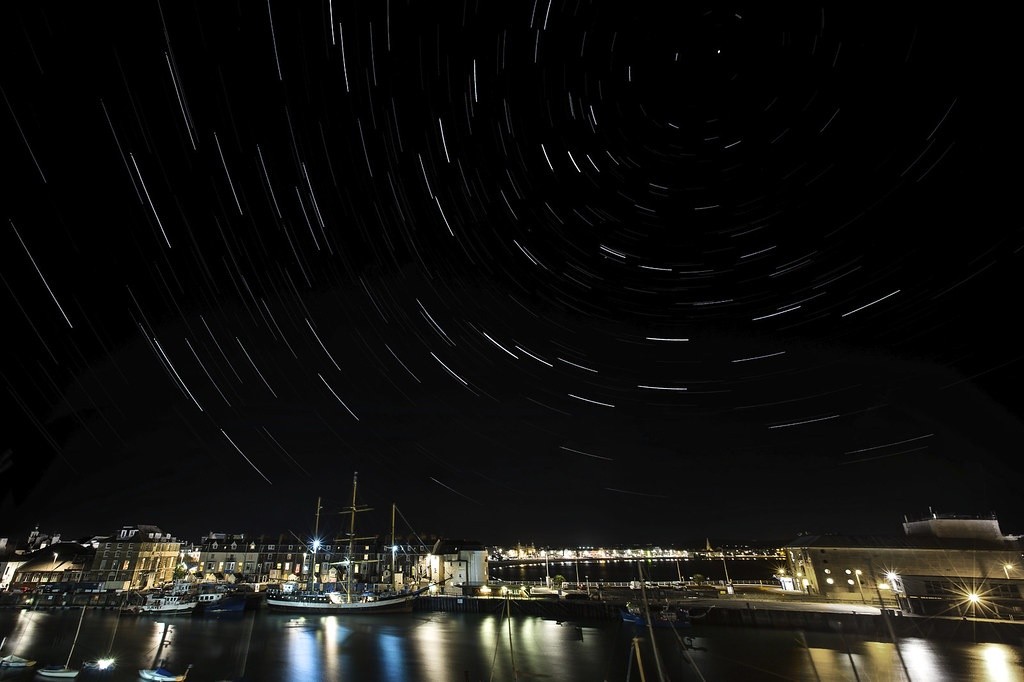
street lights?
[854,569,867,605]
[969,593,979,643]
[887,572,906,613]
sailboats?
[35,606,86,678]
[264,470,454,614]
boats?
[0,653,37,667]
[119,587,198,616]
[196,592,231,607]
[560,583,588,599]
[138,662,192,682]
[618,559,715,630]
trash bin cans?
[727,584,734,594]
